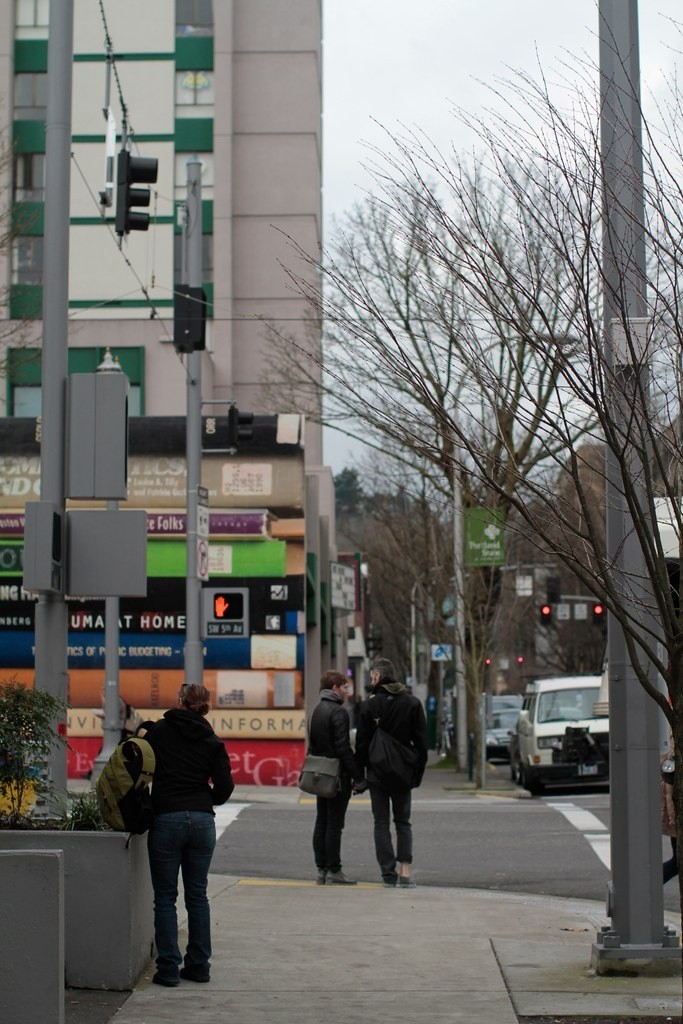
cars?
[483,692,523,763]
[506,722,521,783]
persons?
[143,683,235,988]
[659,733,680,886]
[81,686,143,781]
[351,658,429,888]
[307,670,357,886]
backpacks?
[95,720,155,834]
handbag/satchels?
[363,724,425,788]
[298,754,342,798]
[662,759,677,786]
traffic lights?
[229,405,256,447]
[593,603,605,623]
[115,151,159,236]
[540,604,552,622]
[516,657,525,664]
[485,657,491,666]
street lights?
[453,331,580,783]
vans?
[519,675,610,797]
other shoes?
[316,869,326,885]
[153,972,180,987]
[399,877,417,888]
[180,968,211,982]
[325,869,357,884]
[384,878,395,888]
